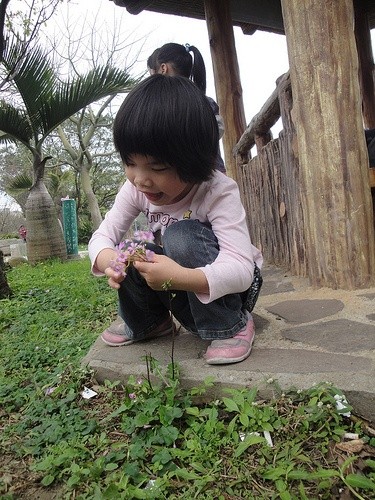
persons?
[88,75,263,366]
[147,43,229,176]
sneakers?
[101,316,176,346]
[206,309,255,364]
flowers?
[107,230,155,278]
